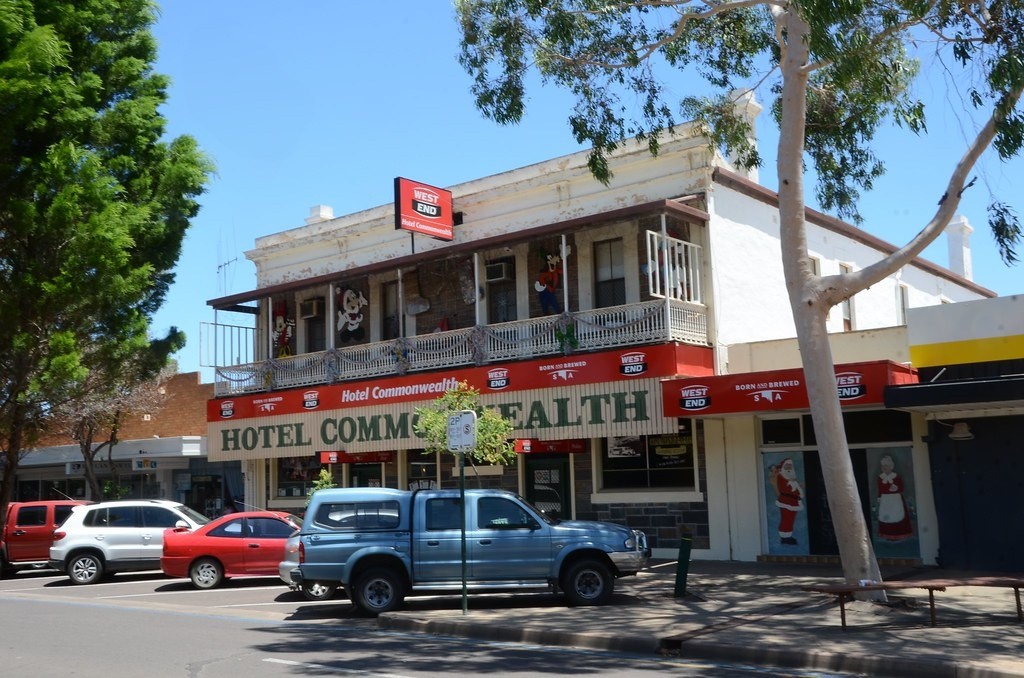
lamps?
[933,412,974,440]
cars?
[278,528,343,601]
[160,511,303,590]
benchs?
[800,577,1024,626]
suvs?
[48,499,253,585]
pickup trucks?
[290,487,652,613]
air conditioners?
[485,262,514,283]
[300,300,323,320]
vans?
[0,498,95,569]
